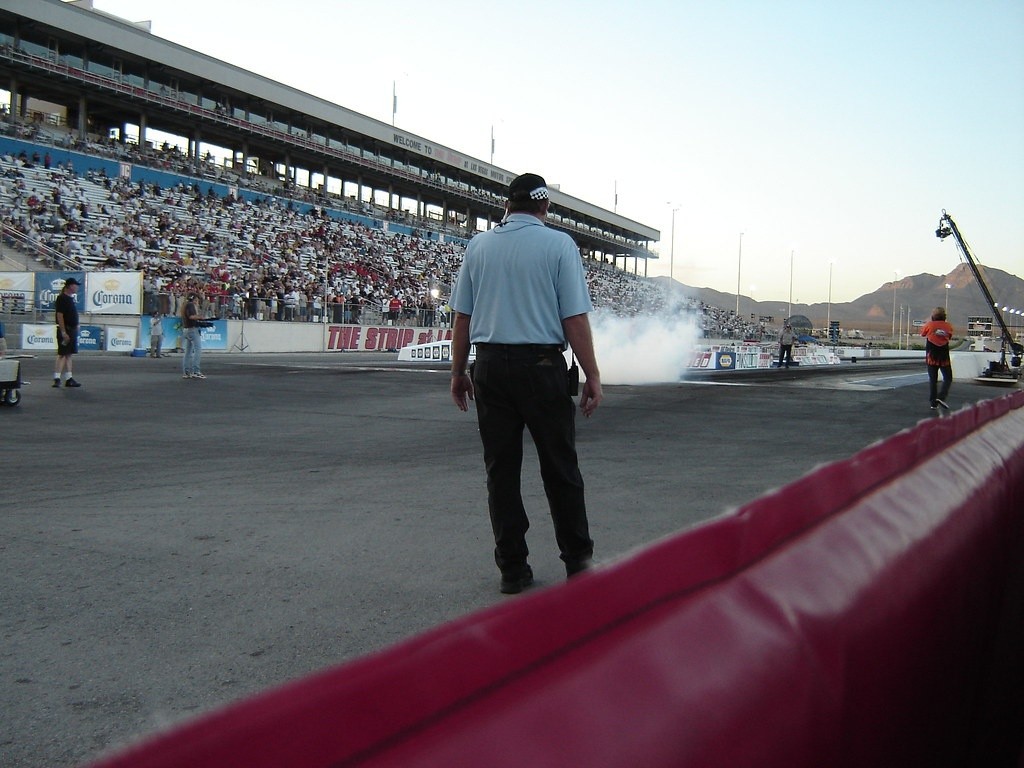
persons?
[448,173,602,594]
[0,36,873,389]
[922,307,954,411]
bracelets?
[61,332,66,335]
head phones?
[192,291,195,299]
